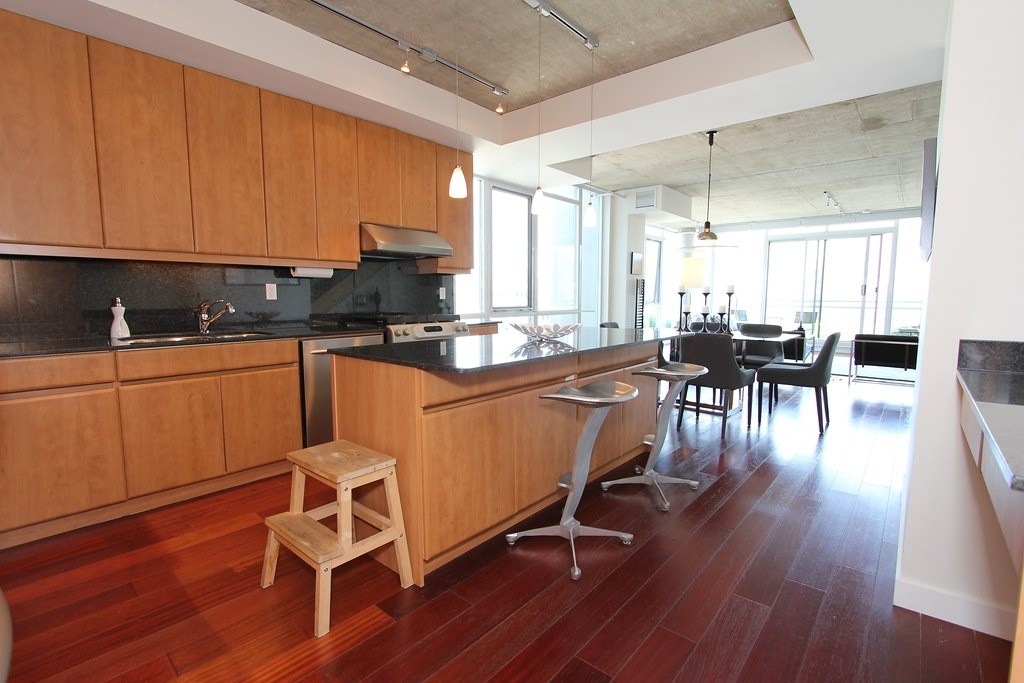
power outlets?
[265,284,277,301]
[440,288,446,299]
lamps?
[531,8,549,217]
[584,47,600,229]
[449,49,468,199]
[698,129,717,241]
[402,51,410,72]
[495,94,504,113]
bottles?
[111,307,132,347]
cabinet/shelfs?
[260,86,361,269]
[113,339,302,515]
[0,8,104,257]
[260,439,415,638]
[437,142,473,276]
[357,119,437,232]
[87,33,266,266]
[0,354,126,550]
[327,354,696,589]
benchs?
[848,334,919,387]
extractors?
[360,223,453,262]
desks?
[712,331,801,415]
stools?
[601,362,710,511]
[504,379,640,580]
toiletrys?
[108,295,131,338]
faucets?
[192,297,236,334]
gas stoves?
[308,312,468,343]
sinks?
[117,331,282,349]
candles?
[703,284,710,293]
[719,305,727,313]
[727,285,735,293]
[678,284,685,293]
[683,305,690,312]
[701,305,709,313]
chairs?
[740,323,785,414]
[676,332,755,441]
[757,332,840,434]
[599,322,619,328]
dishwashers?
[302,334,383,448]
